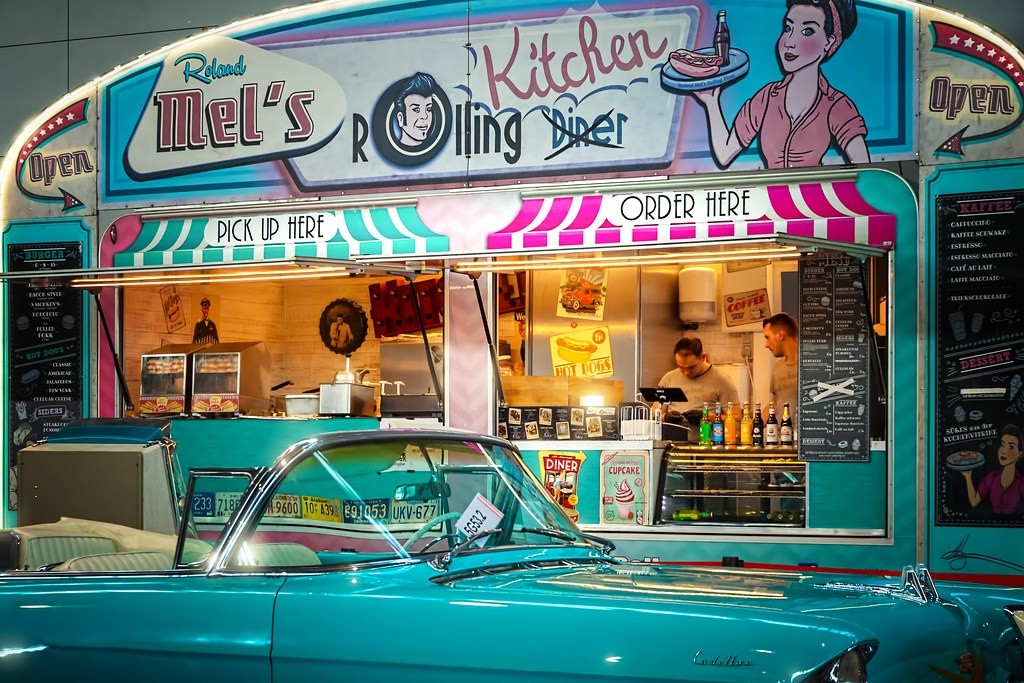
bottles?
[766,511,805,523]
[698,402,712,445]
[766,402,778,445]
[781,402,794,445]
[724,401,736,445]
[752,402,764,446]
[671,509,713,521]
[711,402,724,445]
[740,401,753,445]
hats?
[200,298,211,308]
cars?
[662,467,803,519]
[0,429,1024,683]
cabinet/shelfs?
[653,442,806,528]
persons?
[329,313,353,348]
[763,313,803,512]
[652,338,742,515]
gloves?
[664,411,684,425]
[682,410,703,426]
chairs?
[11,527,119,572]
[234,542,324,570]
[47,549,170,576]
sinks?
[270,395,286,413]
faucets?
[271,380,294,391]
